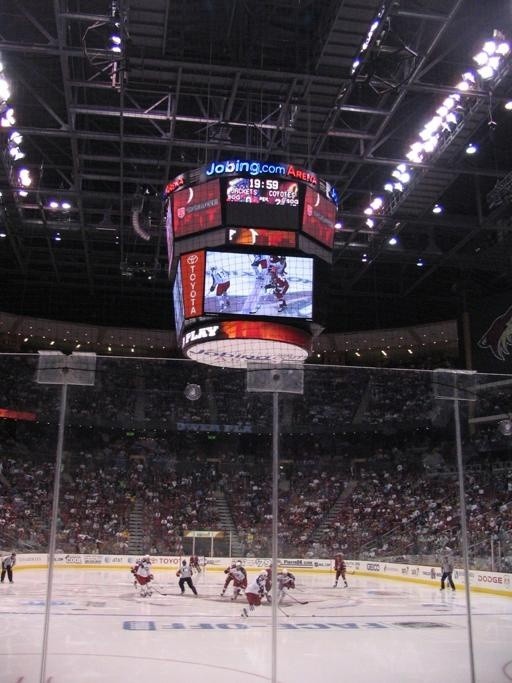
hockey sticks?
[344,570,355,575]
[278,607,296,617]
[284,592,309,604]
[249,288,272,314]
[151,586,167,595]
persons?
[0,434,512,573]
[333,553,348,587]
[441,556,455,590]
[221,559,295,611]
[131,555,154,596]
[176,555,202,597]
[206,253,290,317]
[1,551,16,582]
